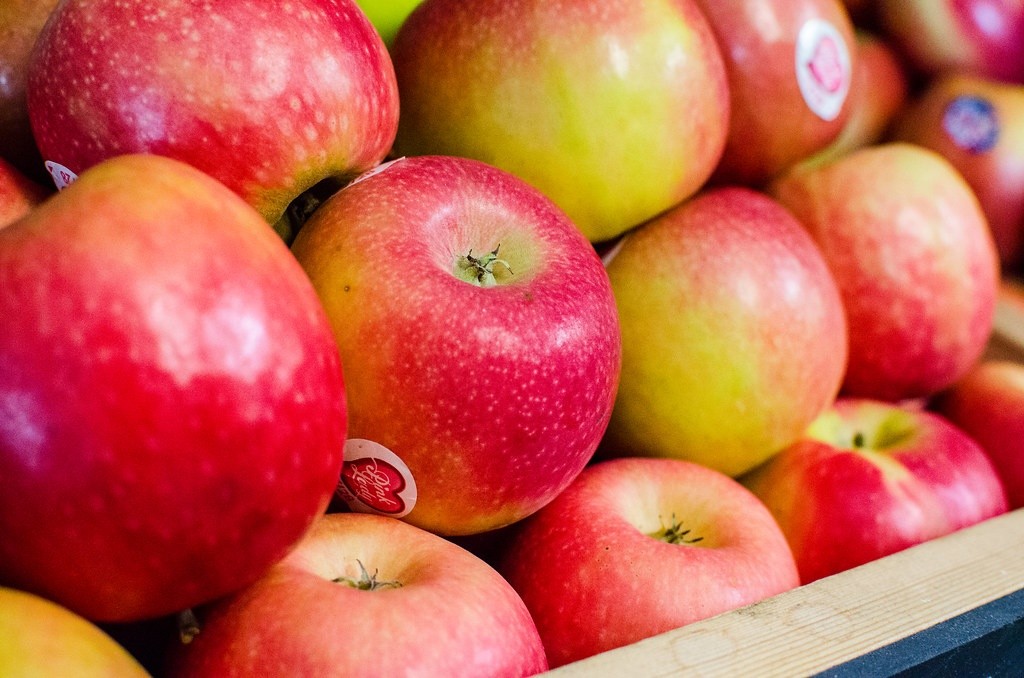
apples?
[0,0,1024,678]
